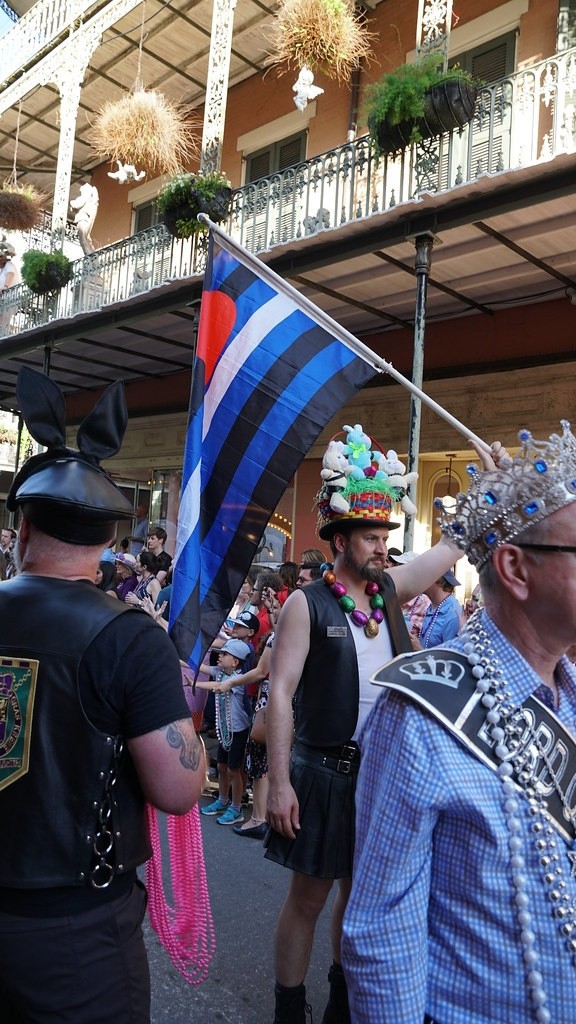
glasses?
[299,577,312,582]
[252,587,260,592]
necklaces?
[146,798,216,986]
[134,573,152,594]
[463,606,575,1024]
[215,667,241,751]
[417,590,454,649]
[407,595,420,622]
[318,562,384,637]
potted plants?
[21,250,71,294]
[364,52,486,158]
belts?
[295,743,360,776]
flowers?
[155,169,230,215]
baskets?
[317,431,392,523]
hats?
[211,639,251,662]
[388,551,419,565]
[100,549,116,565]
[112,553,141,576]
[444,567,461,586]
[319,518,401,541]
[277,590,288,606]
[16,459,138,544]
[388,548,403,556]
[227,610,260,629]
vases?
[162,187,231,237]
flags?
[166,229,386,695]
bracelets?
[4,285,8,289]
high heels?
[233,822,268,839]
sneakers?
[201,800,230,815]
[212,786,249,807]
[216,807,244,824]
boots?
[322,965,350,1024]
[274,991,312,1024]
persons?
[0,458,207,1024]
[69,183,100,276]
[337,416,574,1024]
[263,439,516,1024]
[107,160,145,185]
[130,504,149,556]
[0,255,21,338]
[294,70,314,113]
[0,527,467,839]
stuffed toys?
[320,424,419,516]
